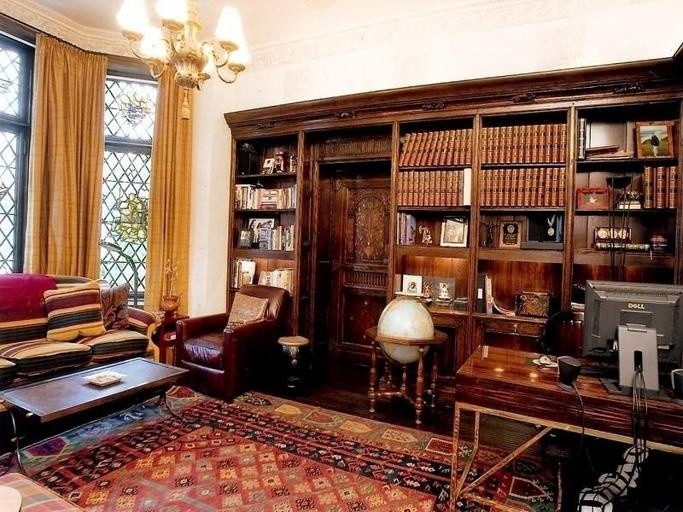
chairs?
[174,284,292,402]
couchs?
[0,274,159,389]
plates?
[81,370,128,387]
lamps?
[114,0,249,119]
[98,242,138,307]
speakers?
[671,369,683,406]
[557,355,582,391]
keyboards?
[579,363,619,379]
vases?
[159,296,181,312]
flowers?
[165,259,178,297]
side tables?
[153,311,190,364]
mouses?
[540,356,551,364]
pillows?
[0,274,130,340]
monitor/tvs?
[581,280,683,402]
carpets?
[0,384,566,512]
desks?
[451,345,683,512]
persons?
[649,132,659,157]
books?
[394,121,564,317]
[640,166,678,209]
[230,138,295,295]
[584,144,633,161]
[575,118,586,161]
